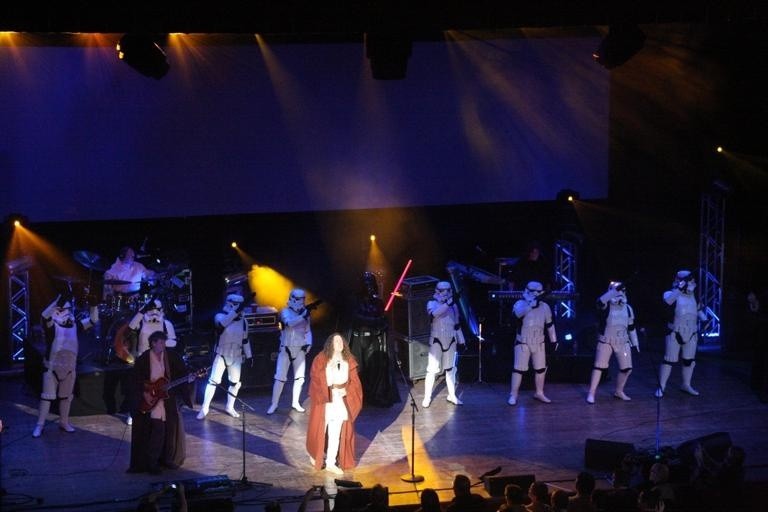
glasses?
[436,287,452,293]
[149,306,164,312]
[526,286,544,294]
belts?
[330,383,347,390]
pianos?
[489,289,579,302]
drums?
[93,317,135,369]
[98,300,113,319]
[111,291,140,314]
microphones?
[336,352,341,370]
[141,237,148,251]
[182,355,189,366]
[640,327,650,343]
[137,255,152,259]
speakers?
[679,432,732,465]
[585,439,634,470]
[484,475,534,497]
[171,496,235,512]
[337,487,388,507]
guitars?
[135,368,209,413]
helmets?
[432,281,455,302]
[675,269,698,294]
[522,280,545,302]
[287,288,307,313]
[54,297,73,319]
[222,293,246,315]
[607,281,629,306]
[143,298,165,321]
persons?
[102,246,154,295]
[125,331,198,475]
[196,294,253,419]
[307,333,364,474]
[507,246,554,289]
[419,488,442,511]
[147,475,392,511]
[422,281,466,407]
[266,288,313,415]
[126,299,178,425]
[654,270,700,399]
[350,272,401,407]
[447,474,487,511]
[31,293,98,438]
[501,446,746,511]
[507,280,559,406]
[586,282,640,403]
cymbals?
[74,250,111,271]
[99,280,131,285]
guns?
[679,268,699,289]
[531,291,549,309]
[616,279,632,291]
[57,281,74,309]
[235,293,257,313]
[140,292,160,314]
[446,287,463,306]
[298,299,322,321]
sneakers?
[290,400,307,413]
[446,393,464,406]
[680,382,700,396]
[532,391,552,404]
[654,385,666,398]
[325,462,345,475]
[421,395,433,408]
[264,402,280,415]
[613,390,633,401]
[58,420,76,433]
[32,422,46,438]
[195,404,210,420]
[223,404,241,419]
[507,390,519,406]
[306,450,316,466]
[585,389,596,404]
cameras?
[171,483,177,489]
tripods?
[189,366,273,497]
